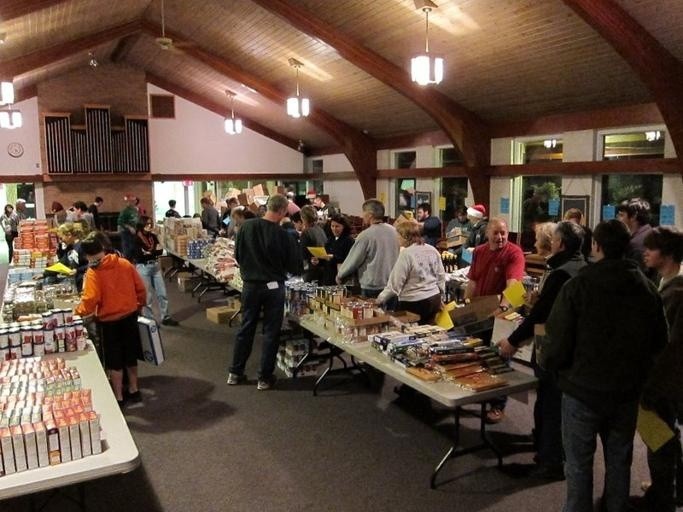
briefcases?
[136,314,165,366]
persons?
[227,189,355,390]
[494,199,683,512]
[336,200,524,422]
[165,195,239,237]
[0,193,161,409]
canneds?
[284,281,318,317]
[0,308,86,360]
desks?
[0,325,143,501]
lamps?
[408,0,445,87]
[284,57,311,121]
[223,88,243,137]
[0,81,23,130]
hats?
[466,205,488,220]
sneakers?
[227,373,247,385]
[257,375,277,390]
[117,387,142,412]
[486,408,505,424]
[527,464,564,480]
[162,315,178,326]
[641,481,652,492]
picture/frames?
[413,190,431,215]
[558,193,589,230]
